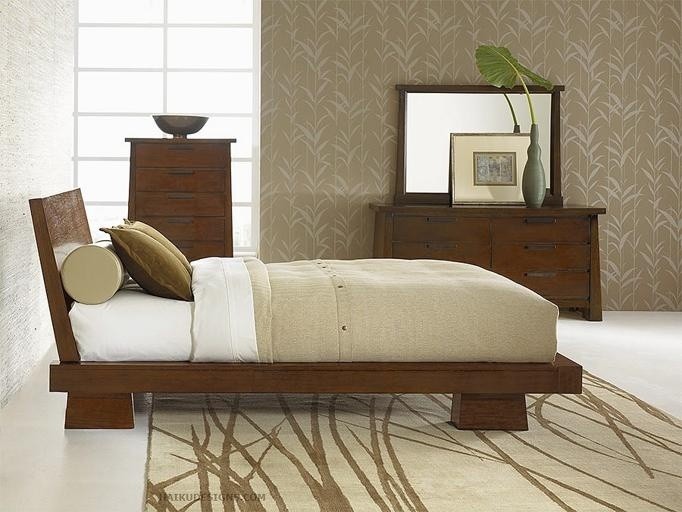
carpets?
[143,370,681,512]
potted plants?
[475,45,555,208]
[504,93,520,133]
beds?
[29,188,582,431]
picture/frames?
[450,133,531,205]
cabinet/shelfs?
[369,204,606,321]
[125,138,236,263]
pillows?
[61,220,192,304]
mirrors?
[396,85,565,204]
